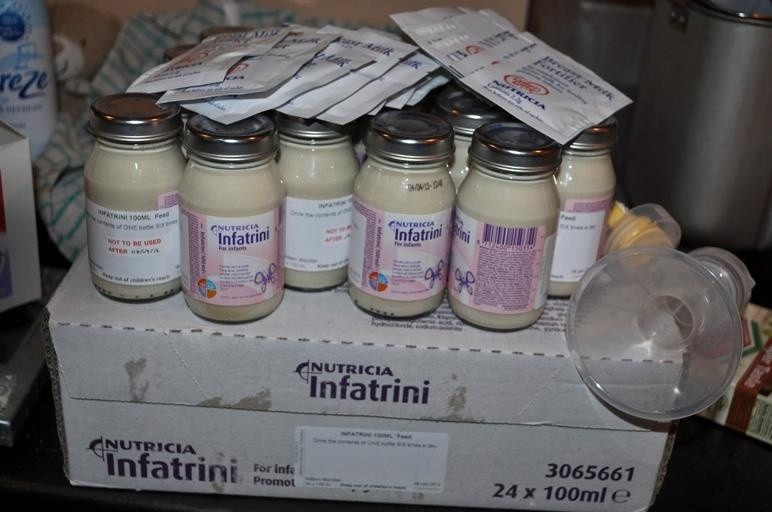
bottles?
[0,2,61,167]
[83,85,617,331]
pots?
[618,0,772,255]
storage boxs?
[39,246,680,510]
[0,119,43,313]
